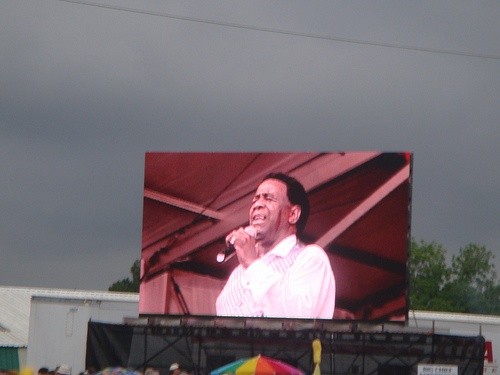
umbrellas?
[207,353,306,375]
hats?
[169,362,182,370]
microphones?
[216,226,257,263]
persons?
[169,363,183,375]
[38,368,49,375]
[215,172,336,320]
